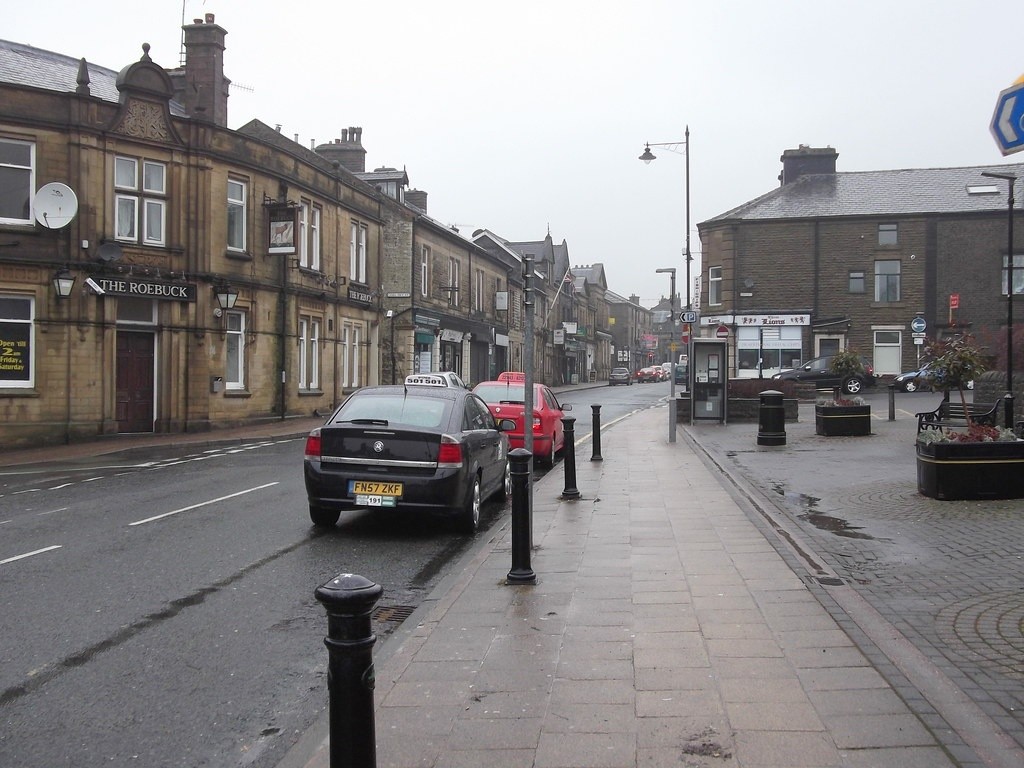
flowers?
[818,396,866,406]
[916,423,1018,444]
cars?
[679,354,688,364]
[637,368,661,384]
[426,372,474,391]
[468,371,573,468]
[891,358,983,392]
[650,366,667,381]
[609,367,634,387]
[302,373,517,532]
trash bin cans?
[757,389,786,446]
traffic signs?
[678,312,697,325]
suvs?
[675,364,687,385]
[772,353,879,396]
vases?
[816,405,871,435]
[916,437,1024,500]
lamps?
[53,267,76,298]
[215,280,239,309]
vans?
[661,362,677,379]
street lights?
[654,267,678,443]
[637,123,694,397]
[981,169,1017,433]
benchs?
[914,397,1001,446]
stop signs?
[680,324,692,344]
[715,326,730,341]
